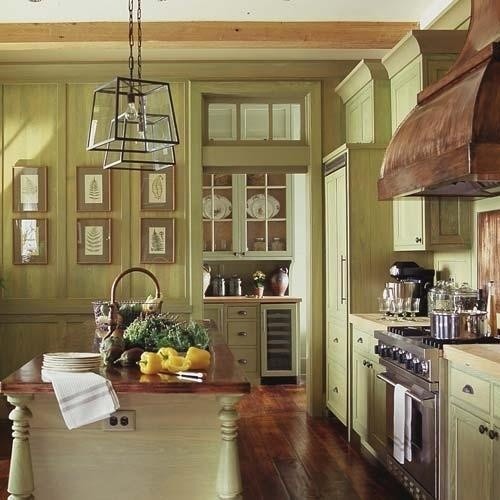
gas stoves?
[373,327,499,382]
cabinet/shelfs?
[334,58,391,145]
[380,29,472,251]
[443,344,500,500]
[201,171,295,261]
[322,144,435,442]
[349,315,387,464]
[202,296,302,385]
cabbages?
[98,337,125,357]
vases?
[253,287,264,296]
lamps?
[85,0,179,153]
[103,0,176,173]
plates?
[202,194,232,219]
[39,352,101,375]
[245,193,281,220]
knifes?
[156,374,203,383]
[158,368,206,379]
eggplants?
[110,326,123,338]
[104,347,142,368]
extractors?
[378,0,500,205]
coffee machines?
[385,261,435,317]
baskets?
[92,268,162,331]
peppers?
[135,345,211,375]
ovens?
[377,364,440,500]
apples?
[118,304,144,316]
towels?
[51,372,120,430]
[393,383,412,465]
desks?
[0,318,250,500]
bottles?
[486,279,498,337]
[426,279,479,319]
[206,237,284,253]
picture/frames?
[140,165,176,212]
[75,166,111,212]
[12,218,47,266]
[12,166,47,213]
[140,218,175,264]
[76,218,112,264]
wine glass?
[378,298,420,323]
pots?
[426,307,487,339]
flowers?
[253,271,266,286]
